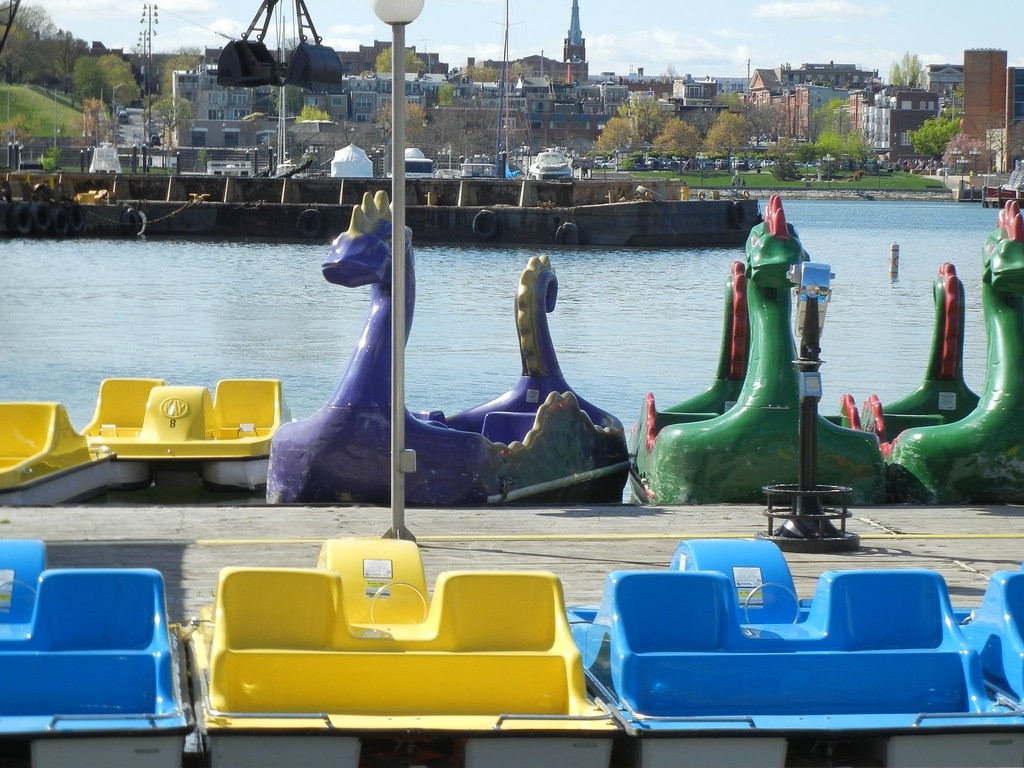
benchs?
[0,402,67,469]
[1,566,173,718]
[207,565,591,714]
[80,377,284,438]
[975,568,1023,697]
[596,567,988,720]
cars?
[114,105,129,124]
[145,114,160,144]
[441,132,899,179]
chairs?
[482,410,535,445]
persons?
[731,175,746,186]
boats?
[1,534,1024,767]
[627,194,886,507]
[266,189,631,510]
[79,378,299,497]
[0,396,159,508]
[883,190,1024,511]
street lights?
[132,144,137,173]
[254,147,258,175]
[176,151,181,174]
[8,140,20,171]
[374,0,426,545]
[245,151,249,160]
[134,4,160,145]
[142,143,147,173]
[268,145,273,172]
[80,147,91,173]
